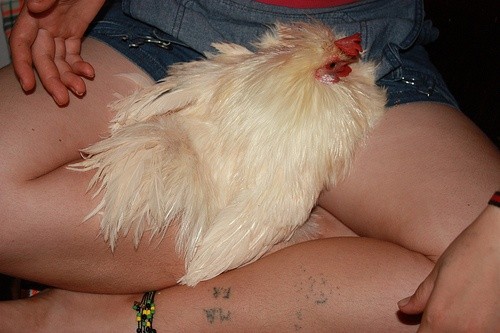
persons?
[1,0,500,333]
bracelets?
[488,190,500,206]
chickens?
[65,18,389,288]
